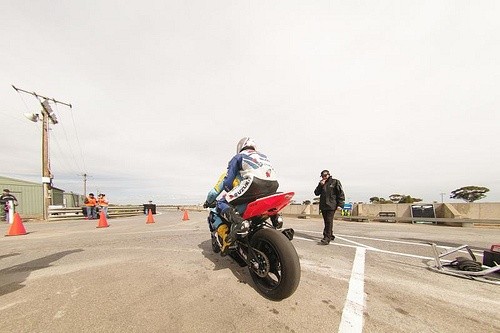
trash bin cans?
[143,203,157,215]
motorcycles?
[204,191,302,302]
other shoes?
[321,239,329,245]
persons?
[217,137,283,243]
[314,170,345,245]
[84,193,98,220]
[98,194,110,219]
[0,188,18,221]
[203,161,247,253]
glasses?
[322,174,328,178]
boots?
[217,224,235,257]
[220,207,249,243]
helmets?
[237,136,259,153]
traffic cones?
[145,208,156,223]
[95,209,111,229]
[183,210,190,220]
[4,211,29,236]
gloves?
[204,201,215,208]
